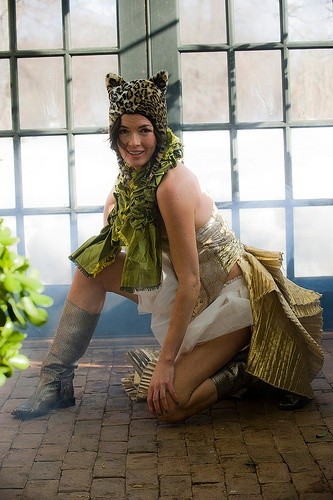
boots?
[209,345,311,411]
[9,296,102,417]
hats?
[105,68,169,182]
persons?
[8,69,325,425]
